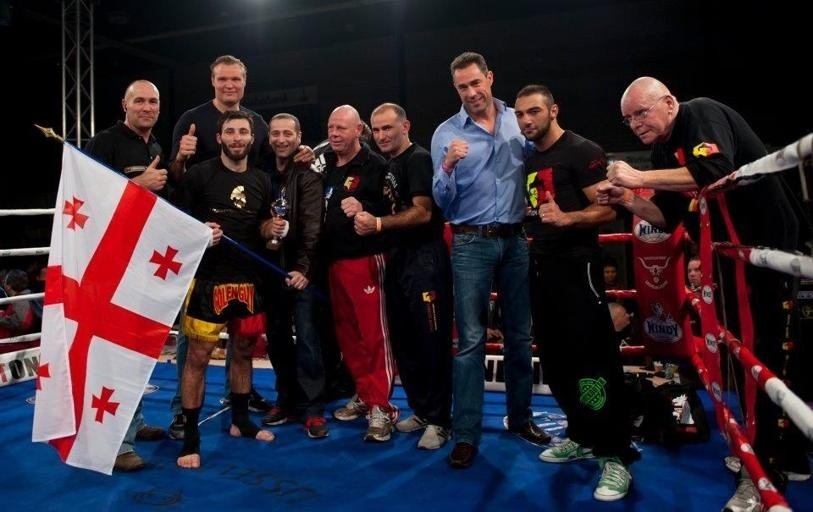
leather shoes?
[136,426,167,441]
[114,451,146,473]
[304,415,329,439]
[263,404,297,426]
[447,442,478,468]
[508,420,551,446]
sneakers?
[332,393,372,421]
[393,414,427,433]
[593,461,633,502]
[721,477,765,511]
[538,438,596,463]
[363,405,394,443]
[416,425,452,450]
[725,456,809,481]
[223,384,273,412]
[168,414,187,440]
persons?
[0,264,44,355]
[83,52,813,511]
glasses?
[622,95,667,125]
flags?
[28,142,214,478]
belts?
[453,223,523,238]
